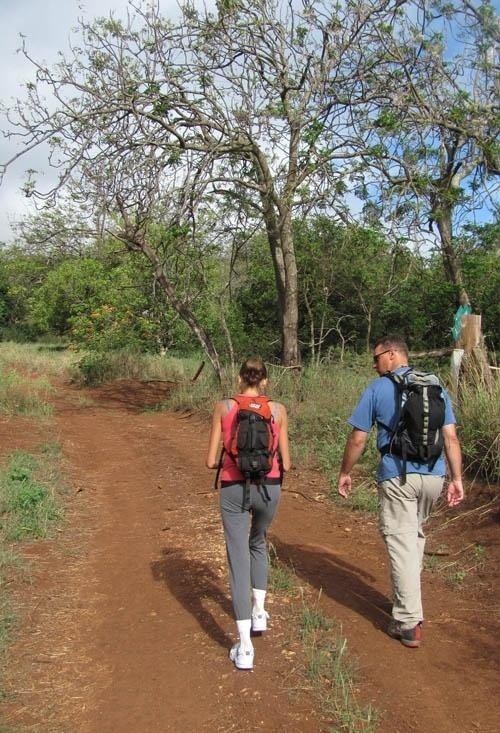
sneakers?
[387,618,422,647]
[251,605,270,632]
[229,642,254,670]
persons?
[205,358,292,670]
[337,335,464,647]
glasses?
[374,348,397,364]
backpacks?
[376,369,446,464]
[228,394,273,479]
